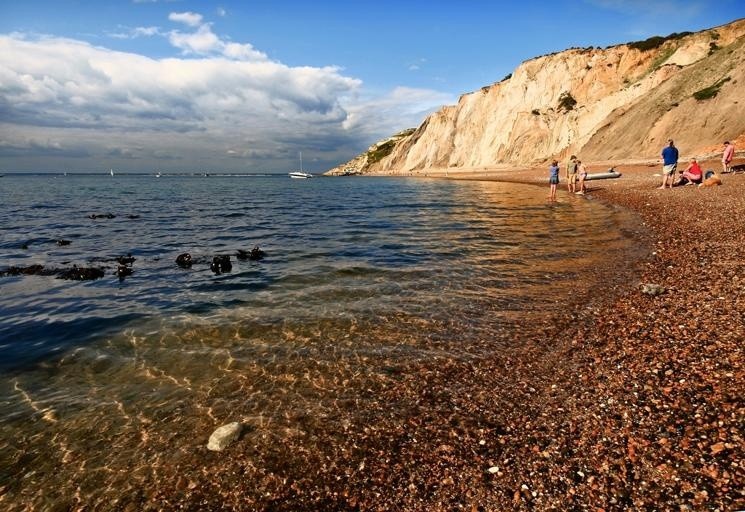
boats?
[575,165,621,180]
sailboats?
[289,152,313,179]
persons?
[720,141,735,174]
[656,139,679,189]
[673,170,688,187]
[575,159,588,195]
[549,159,561,200]
[683,157,702,187]
[566,155,577,193]
